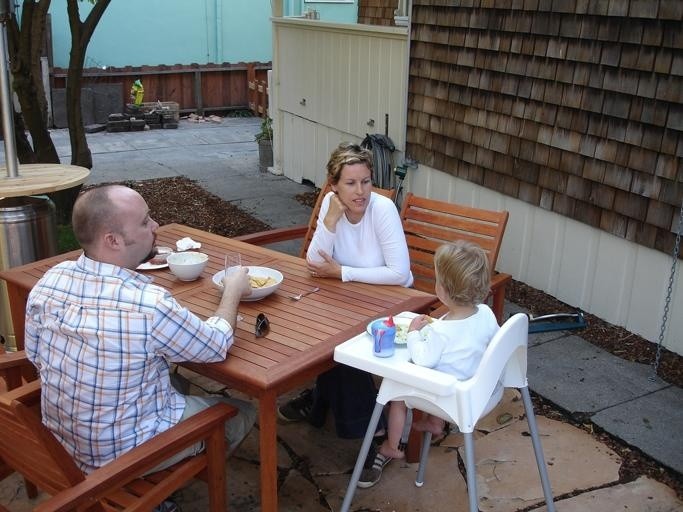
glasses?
[256,312,269,338]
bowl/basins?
[212,265,283,302]
[166,251,209,282]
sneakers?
[356,440,404,489]
[277,386,316,425]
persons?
[22,182,259,511]
[276,139,416,492]
[376,239,501,461]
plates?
[135,247,173,270]
[366,316,413,345]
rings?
[312,270,316,277]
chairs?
[0,377,239,512]
[0,346,39,392]
[401,193,510,464]
[332,311,563,512]
[232,177,396,259]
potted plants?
[254,116,272,172]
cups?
[370,314,396,358]
[224,252,242,278]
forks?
[288,287,320,301]
